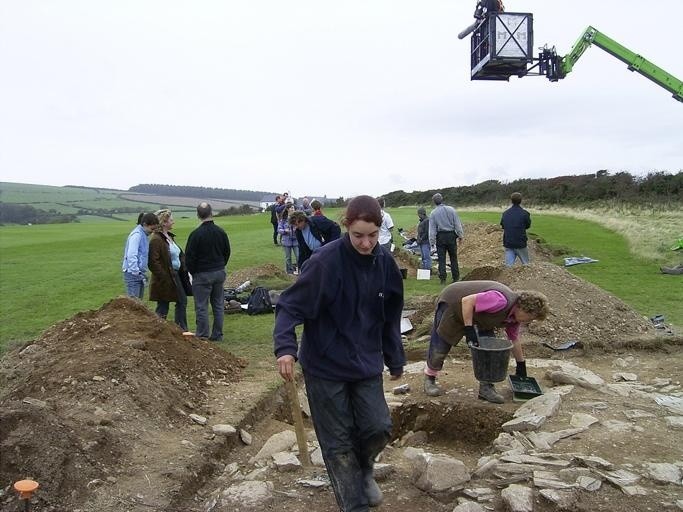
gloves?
[464,326,478,348]
[515,360,527,382]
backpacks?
[247,286,274,316]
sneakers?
[364,478,384,507]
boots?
[424,374,440,397]
[478,382,504,404]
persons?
[473,0,505,60]
[269,192,464,285]
[423,280,550,404]
[271,195,407,512]
[499,191,532,267]
[184,201,232,341]
[121,213,159,302]
[147,208,193,332]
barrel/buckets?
[468,335,514,382]
[400,269,408,279]
